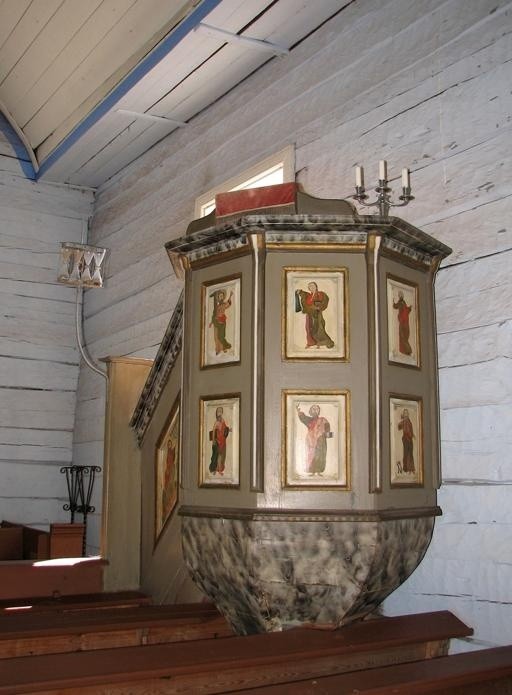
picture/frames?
[197,391,242,490]
[281,266,349,363]
[200,270,241,372]
[386,270,423,370]
[151,387,182,558]
[389,390,426,490]
[281,387,352,493]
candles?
[354,157,409,190]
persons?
[392,289,413,357]
[296,401,335,481]
[208,406,232,477]
[206,288,234,357]
[397,407,417,476]
[161,439,177,493]
[294,280,334,350]
